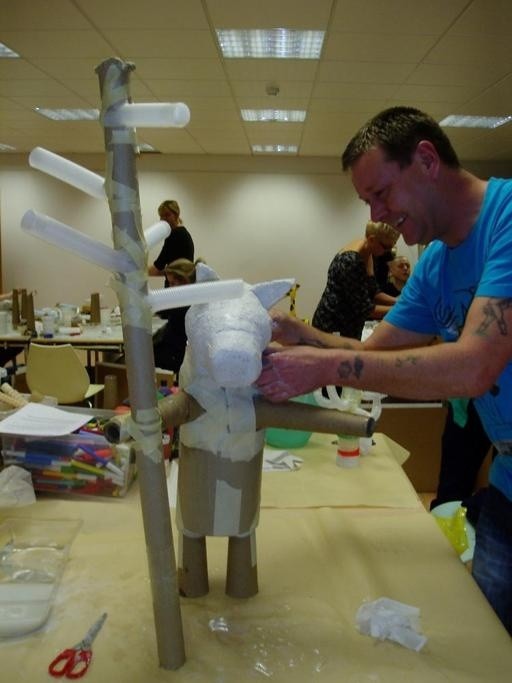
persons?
[256,105,511,634]
[384,256,411,297]
[146,200,195,289]
[153,257,206,371]
[311,217,400,400]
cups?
[0,303,121,338]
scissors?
[48,613,107,679]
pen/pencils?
[1,418,137,497]
